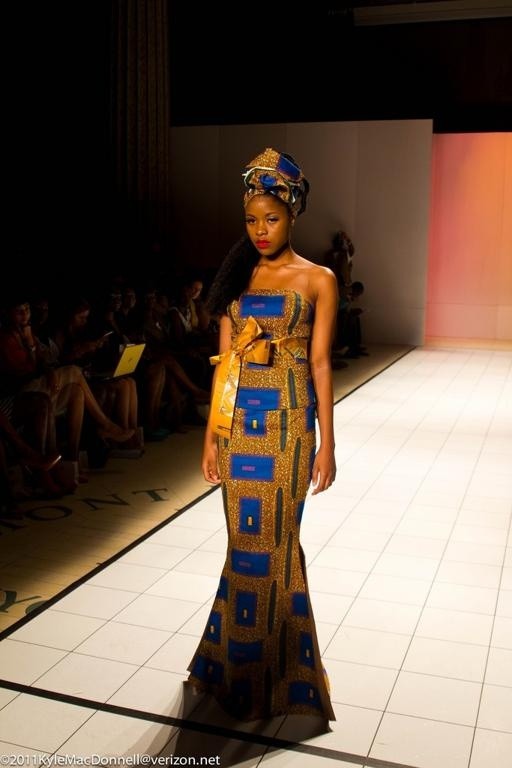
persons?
[186,148,340,721]
[0,230,370,520]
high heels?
[99,429,136,445]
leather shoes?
[30,464,75,496]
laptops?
[92,342,146,380]
